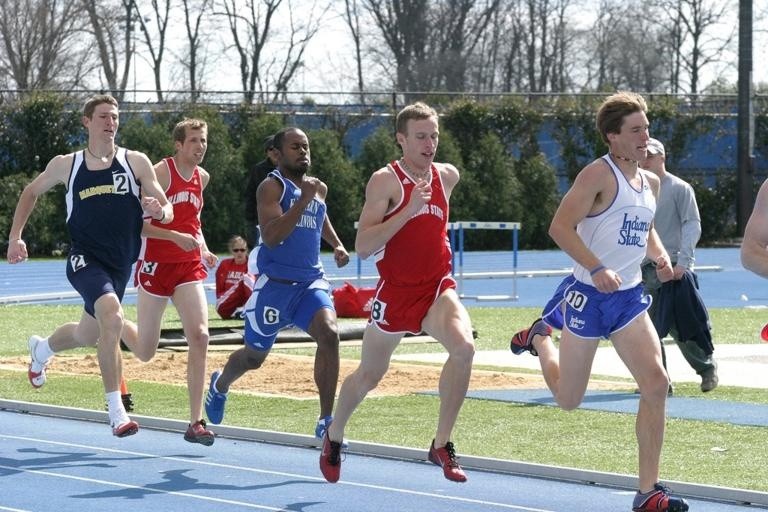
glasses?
[232,248,246,252]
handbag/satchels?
[334,281,377,318]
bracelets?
[587,263,606,279]
[151,210,171,226]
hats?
[646,137,666,156]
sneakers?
[319,426,347,483]
[112,421,139,438]
[105,393,135,413]
[184,419,214,446]
[428,438,468,482]
[635,385,673,396]
[27,335,48,387]
[510,319,552,356]
[632,484,689,511]
[702,361,718,393]
[315,415,349,449]
[204,371,228,424]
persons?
[213,235,256,321]
[737,168,768,346]
[204,125,351,455]
[508,92,690,512]
[318,97,476,486]
[120,116,217,447]
[638,137,720,398]
[5,94,176,437]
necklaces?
[608,149,642,167]
[85,141,114,167]
[398,155,431,180]
